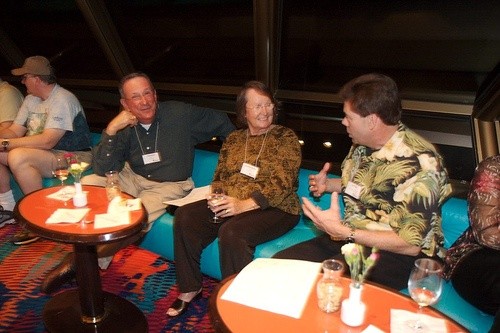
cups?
[52,156,68,181]
[72,192,88,207]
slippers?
[165,287,202,318]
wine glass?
[206,182,224,224]
[407,257,443,333]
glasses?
[245,103,275,111]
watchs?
[345,227,356,243]
[2,137,10,151]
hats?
[11,56,53,76]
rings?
[225,209,228,214]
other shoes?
[41,252,77,293]
[0,204,16,228]
[13,230,41,244]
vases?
[73,182,87,207]
[341,284,366,327]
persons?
[444,155,500,319]
[0,78,27,138]
[270,73,455,293]
[40,73,237,293]
[0,56,95,245]
[166,81,301,317]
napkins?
[107,196,142,212]
[390,308,447,333]
[46,207,91,224]
[93,210,130,228]
[47,185,89,201]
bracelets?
[252,200,256,210]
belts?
[77,148,91,151]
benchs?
[11,133,496,333]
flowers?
[65,153,90,184]
[341,241,378,288]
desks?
[209,270,468,333]
[14,185,149,333]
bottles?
[316,259,344,313]
[105,171,121,200]
